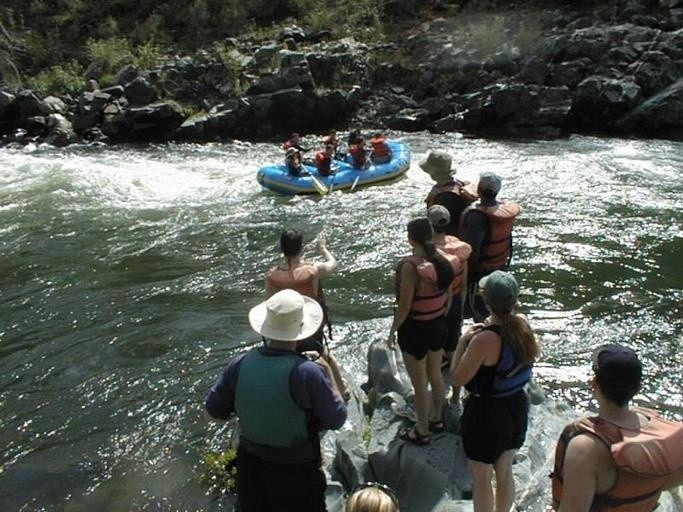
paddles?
[349,150,374,193]
[301,163,328,195]
[329,145,342,193]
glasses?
[348,478,400,509]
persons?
[348,121,366,146]
[285,147,302,177]
[418,151,478,236]
[325,129,341,159]
[205,288,348,512]
[266,229,351,403]
[351,136,369,169]
[461,174,519,323]
[386,214,459,448]
[316,145,336,176]
[284,133,307,154]
[450,270,536,512]
[370,132,392,164]
[552,345,683,512]
[390,205,468,423]
[349,481,399,512]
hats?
[285,147,299,158]
[248,287,323,342]
[480,170,503,197]
[426,204,452,227]
[418,148,458,180]
[591,342,643,397]
[478,269,520,307]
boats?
[256,140,411,195]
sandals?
[426,420,446,433]
[399,427,432,447]
